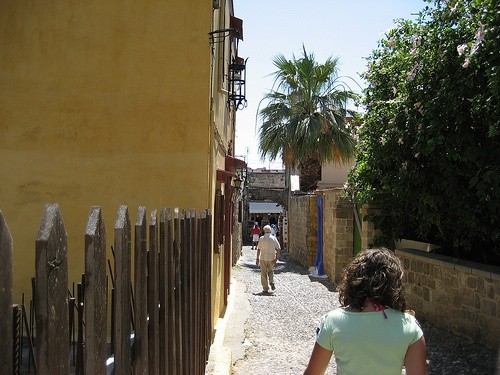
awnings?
[248,201,284,213]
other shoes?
[255,247,257,250]
[251,248,254,250]
[262,291,268,293]
[271,283,276,290]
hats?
[263,225,271,233]
[255,222,258,225]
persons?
[251,222,261,250]
[256,225,281,293]
[270,224,279,239]
[303,247,427,375]
